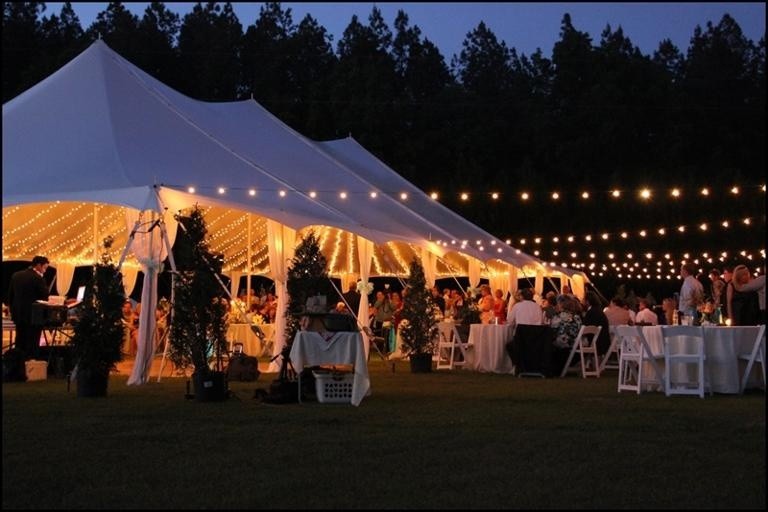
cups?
[681,316,689,326]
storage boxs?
[25,360,49,382]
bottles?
[672,309,678,325]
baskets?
[312,371,354,404]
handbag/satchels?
[191,370,227,401]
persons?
[124,299,168,355]
[479,286,678,357]
[375,286,471,356]
[681,262,767,325]
[3,257,50,348]
[342,281,361,318]
[223,289,277,324]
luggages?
[227,343,260,382]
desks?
[1,326,76,354]
[289,330,371,407]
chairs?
[660,325,713,398]
[616,325,665,395]
[738,324,766,395]
[436,322,468,370]
[517,324,551,379]
[560,325,602,379]
[599,335,620,372]
